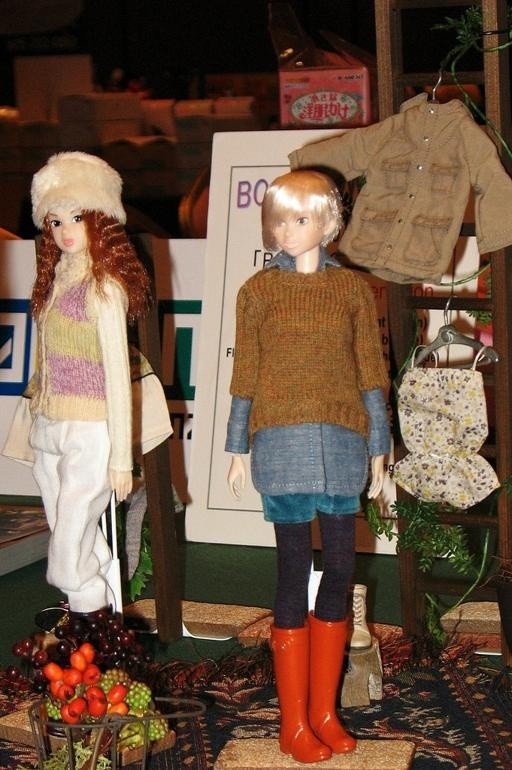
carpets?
[2,618,511,769]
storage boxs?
[277,27,377,128]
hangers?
[405,295,499,369]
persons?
[3,149,177,632]
[224,170,392,763]
